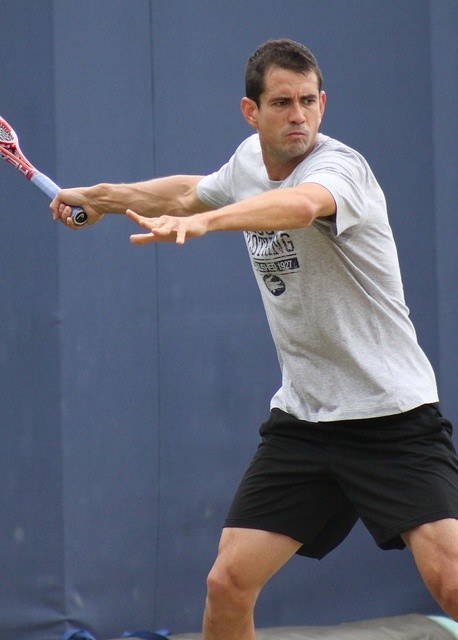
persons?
[49,38,458,640]
